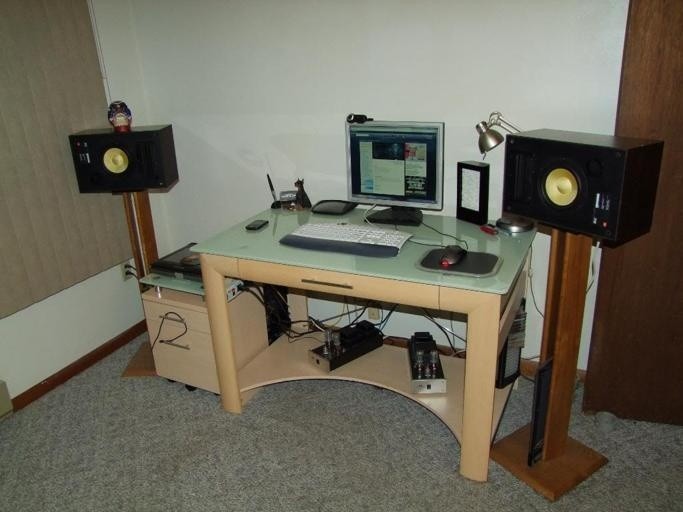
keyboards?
[278,220,415,258]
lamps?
[476,111,534,233]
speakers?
[67,123,180,194]
[502,127,665,249]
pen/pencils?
[267,174,277,201]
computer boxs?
[496,297,527,389]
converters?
[341,327,363,345]
[415,332,430,338]
[413,338,436,353]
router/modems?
[312,199,356,214]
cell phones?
[245,219,269,230]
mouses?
[439,243,467,266]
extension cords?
[227,280,246,303]
[407,337,448,395]
[307,318,385,372]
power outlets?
[121,260,133,283]
[367,301,381,320]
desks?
[189,208,538,482]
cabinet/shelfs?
[139,275,308,396]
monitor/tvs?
[344,119,445,227]
